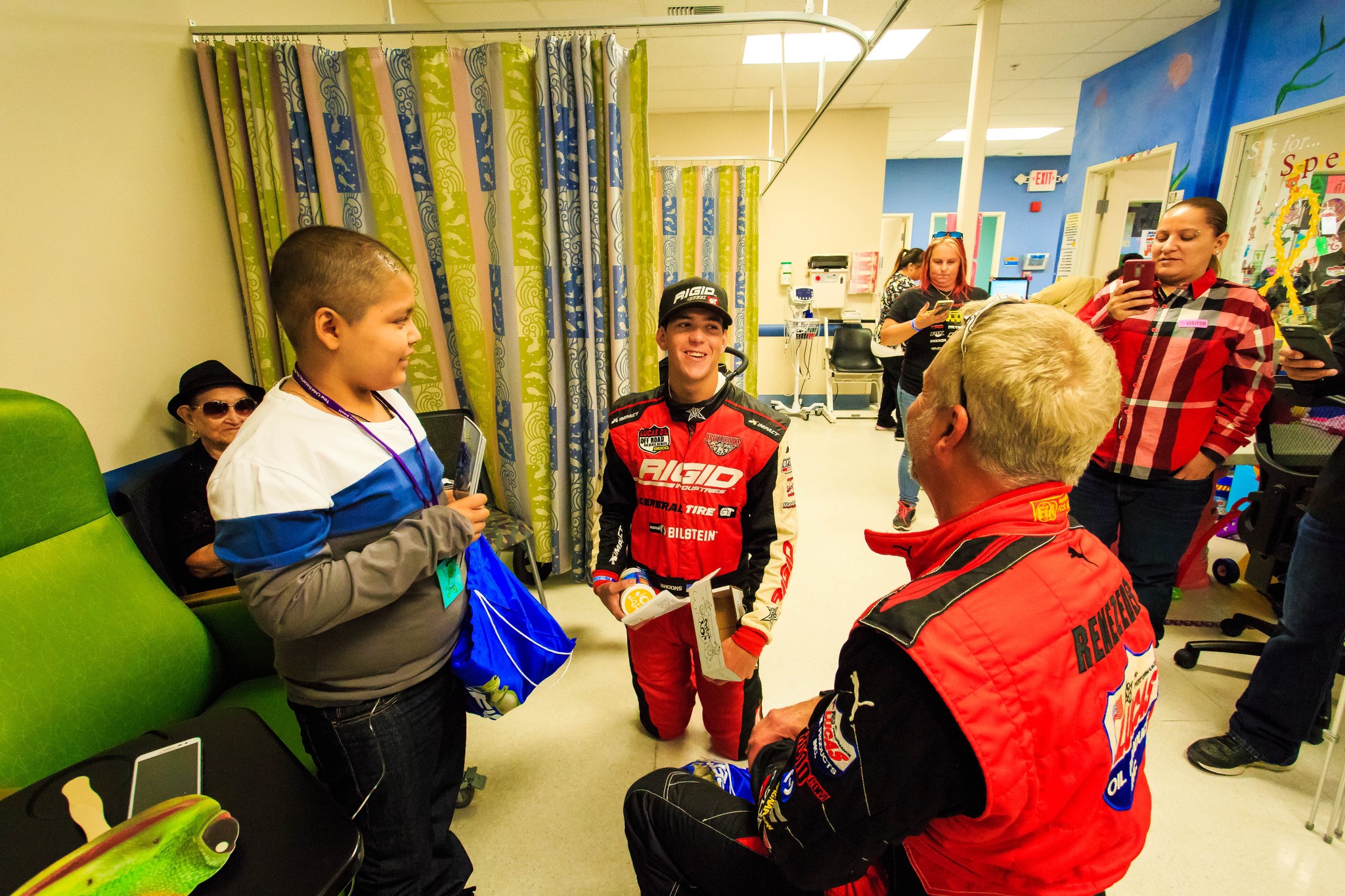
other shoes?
[874,420,898,431]
[894,434,905,442]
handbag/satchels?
[449,533,576,722]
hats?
[168,359,267,424]
[657,276,733,329]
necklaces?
[931,282,954,300]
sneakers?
[892,500,917,531]
[1186,727,1296,776]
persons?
[1065,197,1276,647]
[1284,221,1345,335]
[871,248,925,441]
[1187,319,1345,775]
[1028,265,1123,316]
[623,298,1157,896]
[881,236,992,531]
[207,224,489,896]
[160,360,266,588]
[590,278,798,760]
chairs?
[1173,374,1345,676]
[416,408,548,613]
[821,317,885,424]
[106,459,195,598]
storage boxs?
[689,567,759,682]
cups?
[618,568,657,616]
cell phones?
[1280,325,1343,375]
[127,737,202,822]
[1123,259,1155,309]
[933,300,955,316]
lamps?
[1014,169,1069,191]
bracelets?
[911,318,922,331]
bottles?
[780,262,792,285]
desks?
[0,706,366,896]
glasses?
[931,231,964,245]
[192,398,258,420]
[959,297,1026,438]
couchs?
[0,389,320,806]
